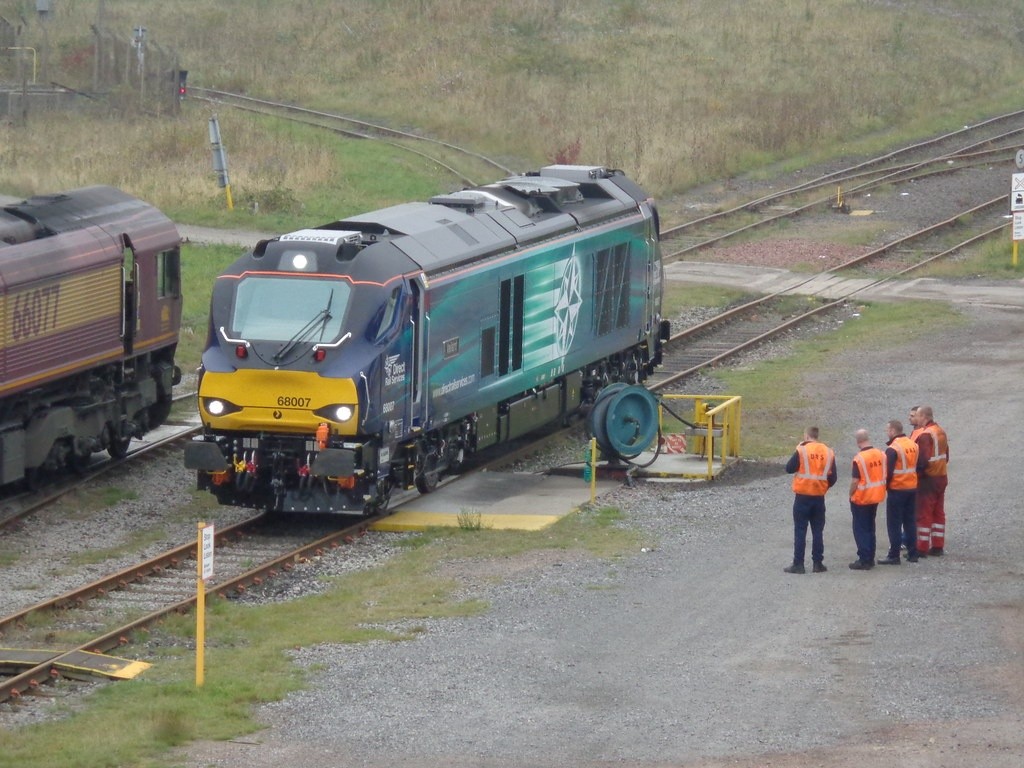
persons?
[783,425,837,573]
[848,405,949,570]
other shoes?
[784,560,805,574]
[812,561,827,572]
[902,551,926,558]
[907,553,918,562]
[848,559,874,570]
[930,547,943,555]
[877,557,901,564]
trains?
[0,190,184,496]
[182,166,672,519]
